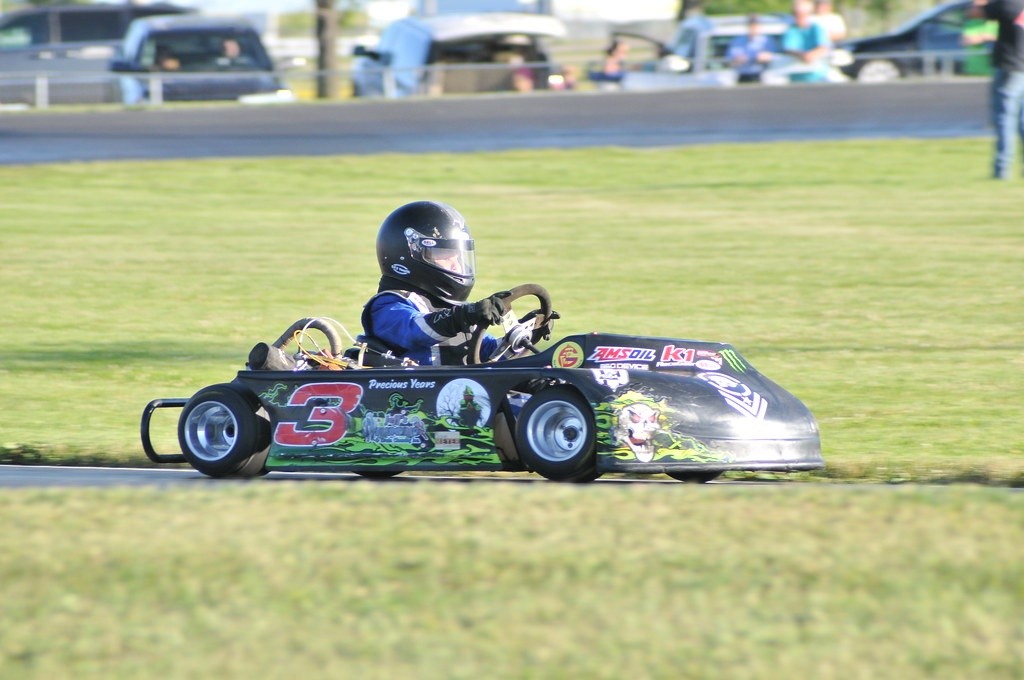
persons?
[510,66,536,90]
[724,0,848,83]
[959,0,1024,179]
[160,39,240,70]
[597,40,627,92]
[361,200,560,366]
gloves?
[451,291,512,326]
[519,308,560,345]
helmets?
[376,201,475,305]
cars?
[592,12,832,76]
[107,13,297,101]
[829,1,1024,84]
[348,12,577,99]
[0,1,206,109]
